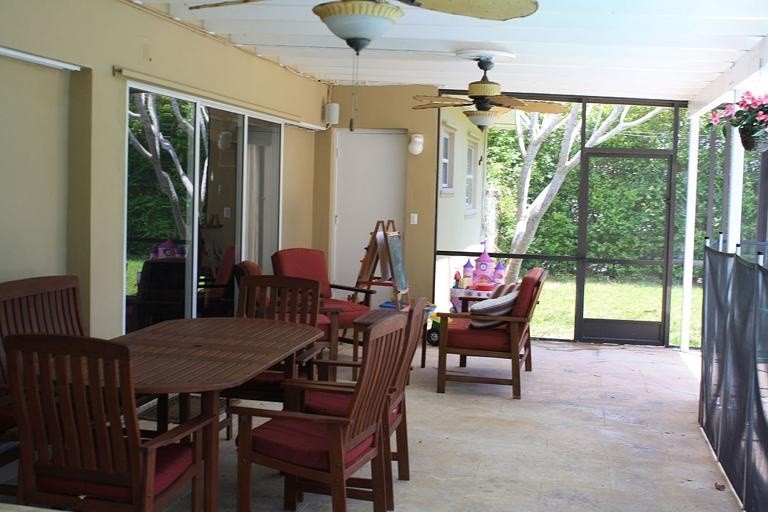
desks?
[353,307,428,376]
[38,317,326,512]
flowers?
[704,90,768,138]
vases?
[737,130,768,152]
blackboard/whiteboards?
[385,230,410,296]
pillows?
[490,282,521,299]
[467,290,522,330]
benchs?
[0,274,92,498]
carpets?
[139,394,240,425]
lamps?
[216,129,235,151]
[309,1,407,58]
[463,58,508,132]
[408,132,426,157]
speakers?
[324,103,339,125]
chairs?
[436,267,549,399]
[220,275,319,440]
[288,297,428,512]
[271,248,374,380]
[234,261,342,380]
[4,335,219,512]
[198,247,235,316]
[226,312,408,512]
[126,256,186,330]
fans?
[407,48,570,133]
[188,0,539,57]
